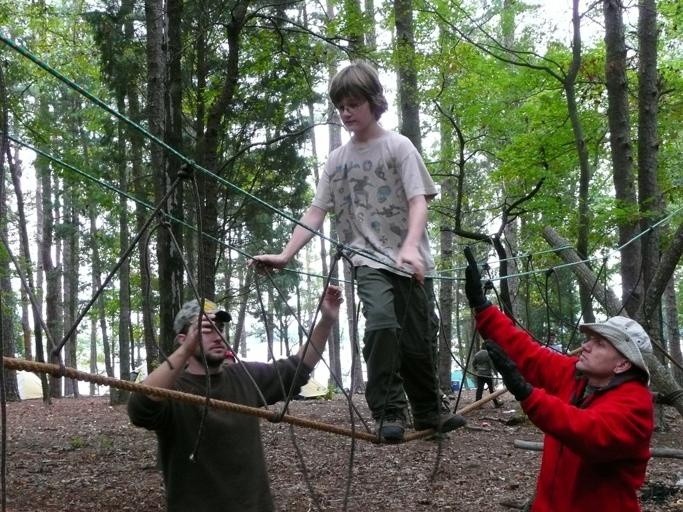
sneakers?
[496,402,503,408]
[476,406,485,410]
[411,410,466,433]
[372,413,404,442]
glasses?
[335,100,367,114]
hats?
[581,318,655,388]
[172,297,232,333]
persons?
[464,246,655,512]
[221,352,235,368]
[542,334,563,356]
[472,343,503,408]
[127,285,344,512]
[248,64,467,442]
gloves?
[463,246,492,312]
[482,339,532,402]
